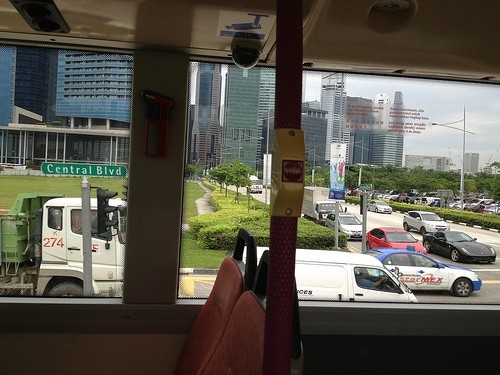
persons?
[357,267,387,289]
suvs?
[246,180,263,194]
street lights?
[305,146,315,186]
[431,122,465,211]
[348,134,364,187]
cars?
[446,198,500,214]
[367,200,392,214]
[325,213,363,241]
[422,231,496,264]
[403,211,450,235]
[362,247,482,297]
[346,188,363,196]
[370,190,441,207]
[366,227,428,257]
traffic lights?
[121,184,128,202]
[96,188,119,241]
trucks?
[0,192,128,297]
[301,186,347,225]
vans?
[242,246,418,303]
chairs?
[173,227,304,375]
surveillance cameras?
[230,39,263,71]
